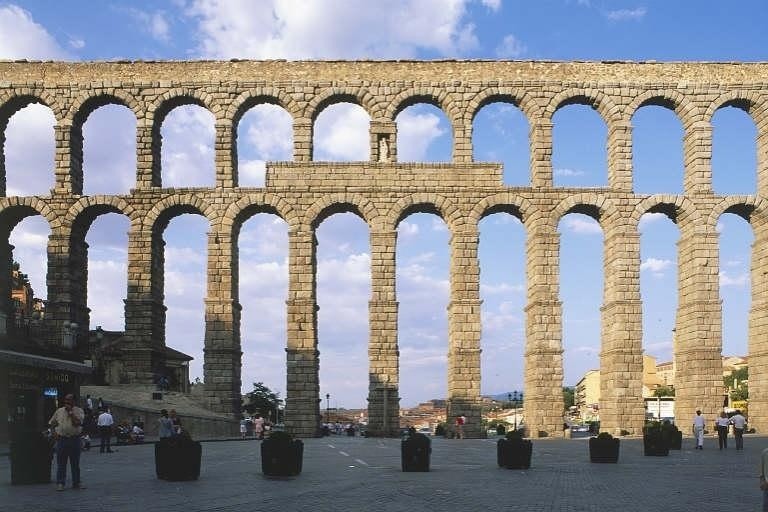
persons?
[47,392,85,490]
[253,414,265,439]
[714,410,730,449]
[460,412,465,424]
[152,408,176,442]
[691,409,706,449]
[168,409,180,435]
[318,418,366,435]
[80,394,144,453]
[760,447,768,511]
[729,409,746,451]
[239,416,249,440]
[453,414,463,439]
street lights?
[657,391,661,422]
[326,393,331,424]
[507,391,523,430]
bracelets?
[68,410,74,415]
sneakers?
[100,448,114,454]
[56,483,64,491]
